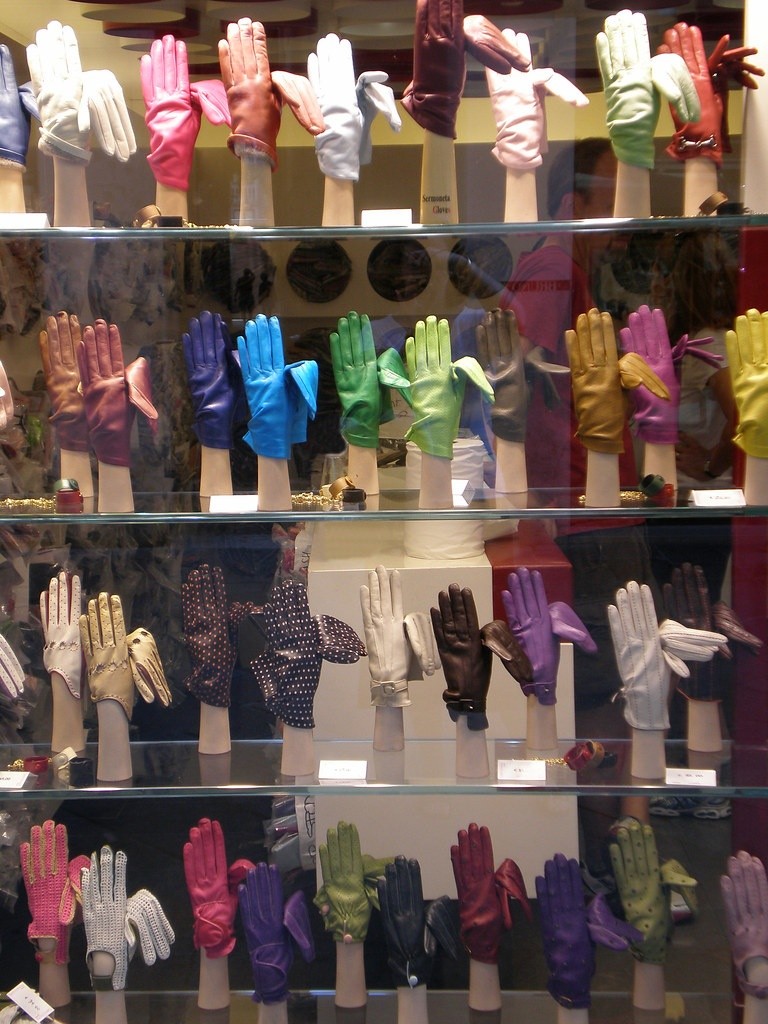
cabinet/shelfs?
[0,213,768,1024]
[307,478,578,900]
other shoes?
[649,797,734,819]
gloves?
[0,0,764,190]
[23,820,768,1011]
[0,564,765,728]
[39,305,768,467]
